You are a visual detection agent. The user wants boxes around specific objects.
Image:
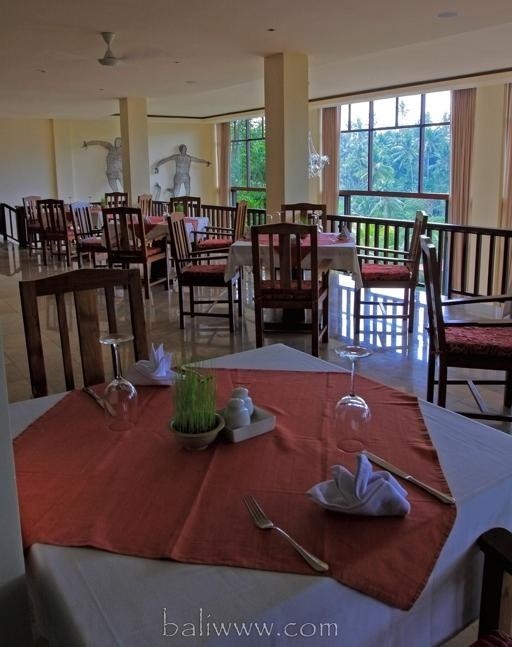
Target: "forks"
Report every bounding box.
[242,494,329,573]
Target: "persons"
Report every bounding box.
[153,144,214,198]
[80,136,124,193]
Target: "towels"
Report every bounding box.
[304,453,410,517]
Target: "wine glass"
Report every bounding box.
[333,345,371,452]
[311,215,321,238]
[314,211,323,233]
[98,333,138,432]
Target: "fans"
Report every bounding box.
[71,31,158,66]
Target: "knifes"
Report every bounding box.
[83,386,116,416]
[364,450,455,505]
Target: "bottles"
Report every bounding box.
[231,387,253,416]
[224,398,250,428]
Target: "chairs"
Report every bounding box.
[469,527,512,647]
